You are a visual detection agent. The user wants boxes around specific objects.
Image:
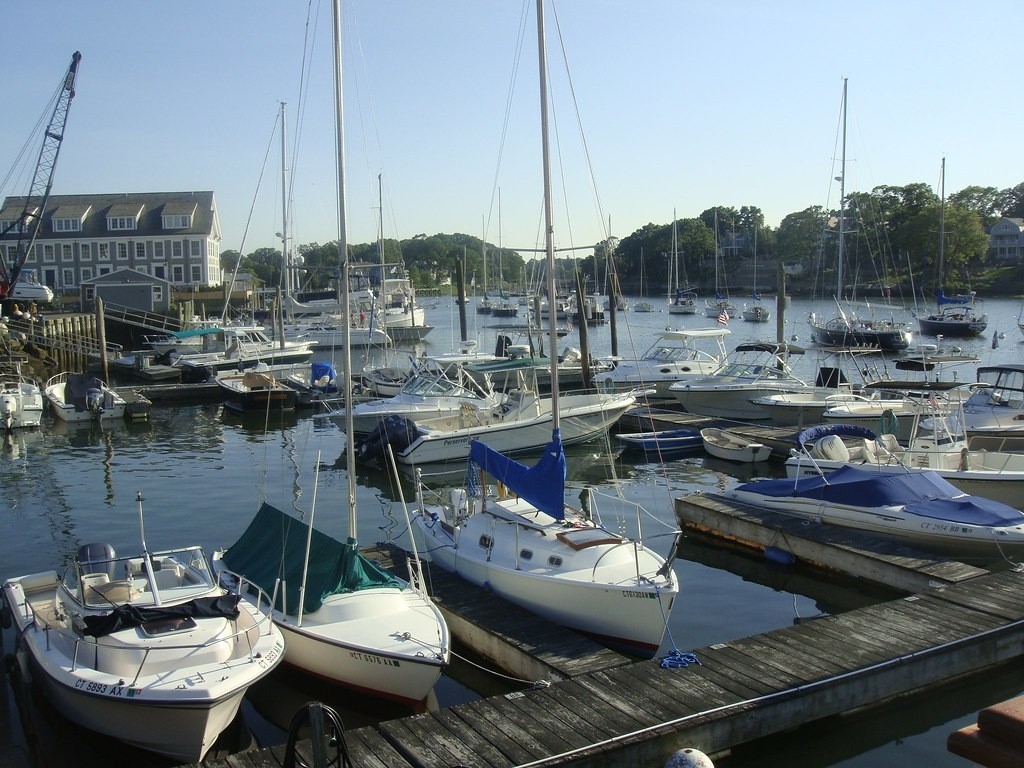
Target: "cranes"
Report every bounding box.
[0,50,83,319]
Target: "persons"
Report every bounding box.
[12,302,39,322]
[350,310,366,326]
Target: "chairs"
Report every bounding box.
[81,573,111,606]
[879,434,905,452]
[146,565,182,590]
[863,438,895,465]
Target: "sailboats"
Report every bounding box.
[139,1,1023,707]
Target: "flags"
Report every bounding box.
[718,310,729,326]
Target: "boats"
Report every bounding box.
[1,488,290,765]
[45,371,128,424]
[0,373,44,429]
